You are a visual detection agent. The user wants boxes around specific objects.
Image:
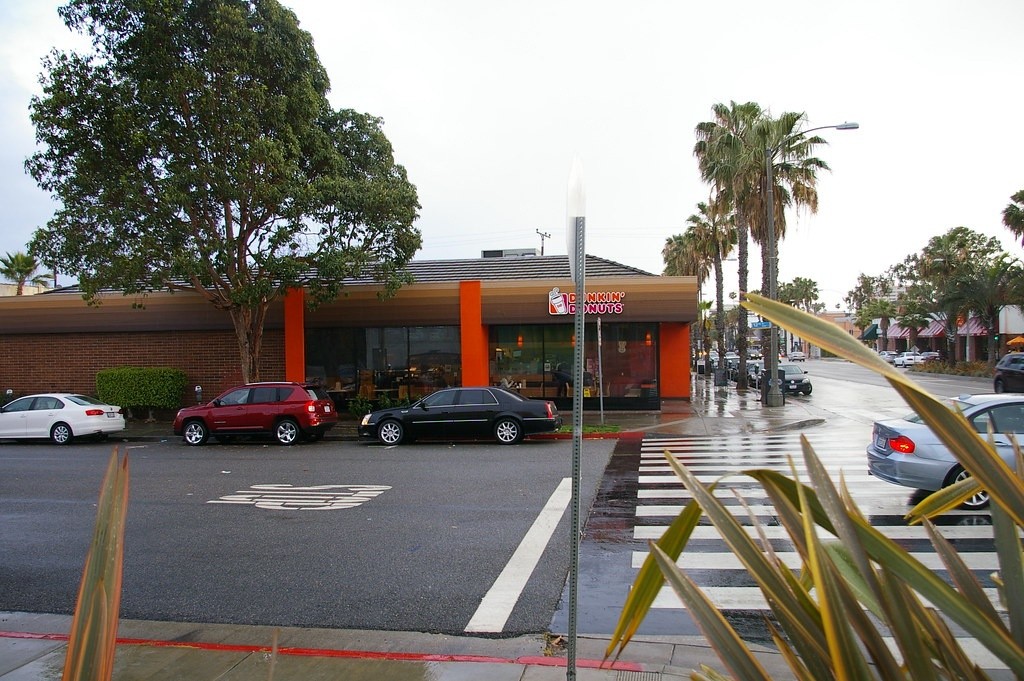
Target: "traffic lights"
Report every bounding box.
[994,335,1000,349]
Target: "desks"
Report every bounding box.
[511,386,526,395]
[372,388,398,392]
[326,389,355,411]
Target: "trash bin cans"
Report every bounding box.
[698,365,704,374]
[714,366,727,385]
[761,369,785,405]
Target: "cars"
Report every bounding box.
[893,351,924,368]
[867,392,1024,510]
[357,386,563,445]
[709,351,765,388]
[778,363,812,396]
[526,370,592,387]
[920,352,945,363]
[746,349,762,360]
[0,393,126,445]
[880,351,898,363]
[788,352,805,362]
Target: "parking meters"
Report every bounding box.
[6,389,13,404]
[194,385,203,405]
[754,363,759,396]
[728,360,732,387]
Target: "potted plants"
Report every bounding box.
[348,393,372,423]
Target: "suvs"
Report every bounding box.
[173,381,338,446]
[993,352,1024,393]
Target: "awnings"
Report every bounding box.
[887,323,923,339]
[957,317,998,337]
[918,320,946,337]
[857,324,878,339]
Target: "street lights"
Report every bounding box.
[934,258,970,361]
[766,123,860,409]
[700,258,738,352]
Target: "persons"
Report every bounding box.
[1008,347,1024,353]
[555,363,569,397]
[500,374,514,388]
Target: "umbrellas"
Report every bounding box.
[1006,336,1024,346]
